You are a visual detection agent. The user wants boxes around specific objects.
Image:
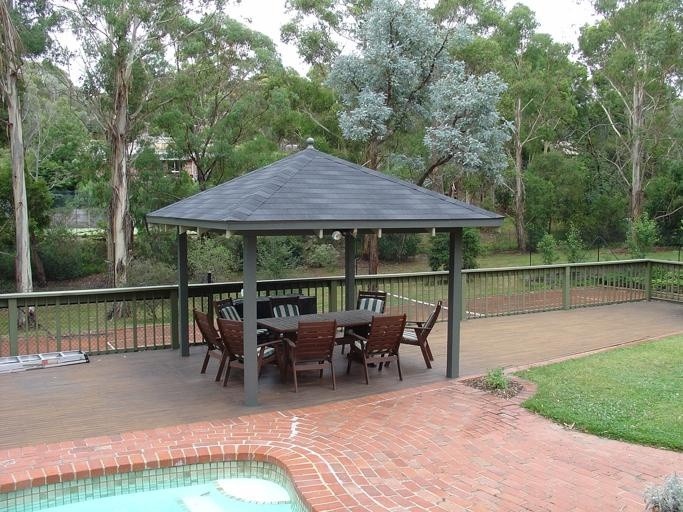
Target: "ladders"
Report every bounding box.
[0,350,89,373]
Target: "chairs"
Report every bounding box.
[191,290,441,394]
